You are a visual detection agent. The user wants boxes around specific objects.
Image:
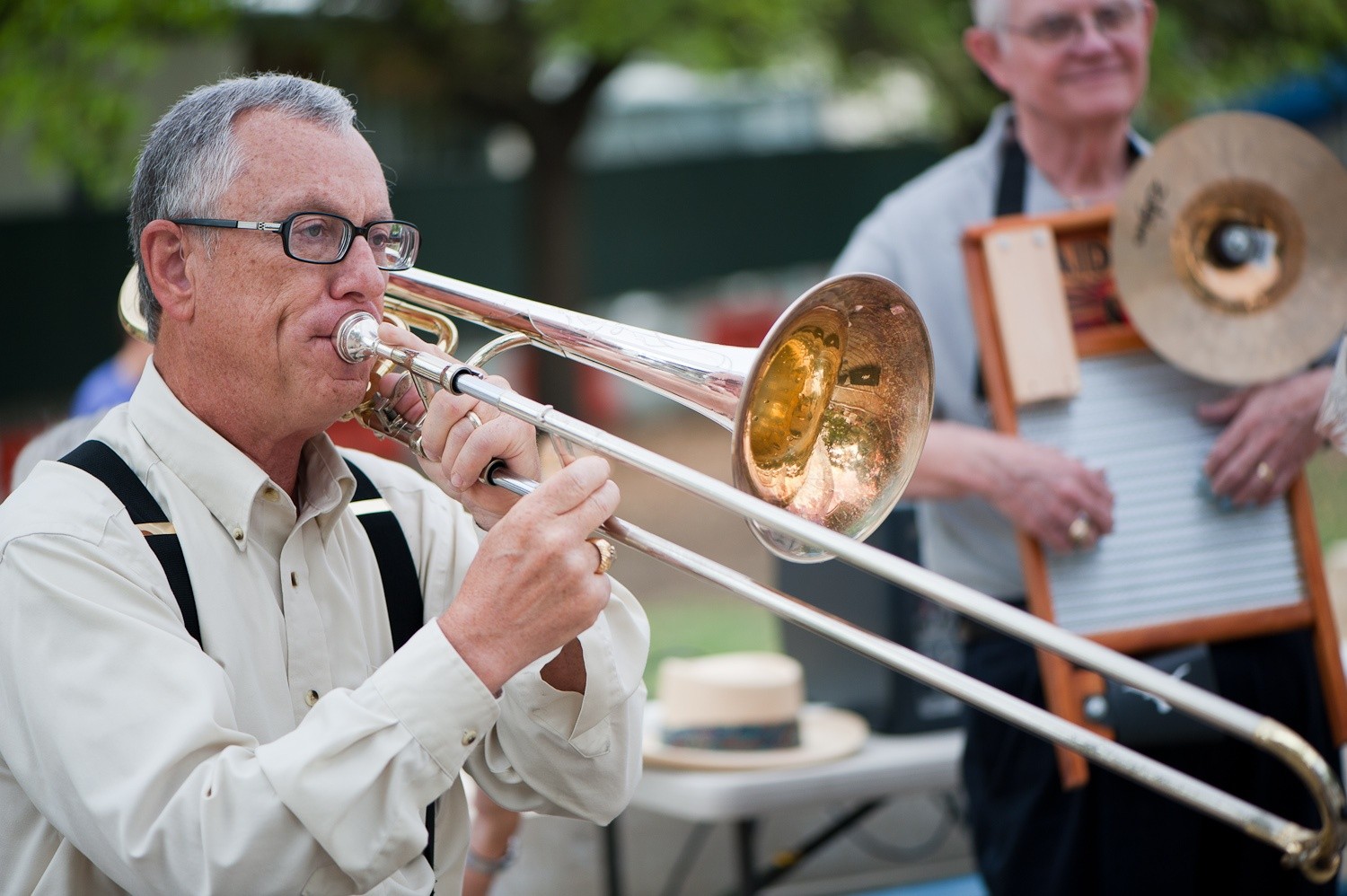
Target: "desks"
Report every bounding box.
[601,726,976,896]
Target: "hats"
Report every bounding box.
[637,648,873,775]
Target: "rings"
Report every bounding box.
[1255,461,1277,486]
[1065,509,1092,545]
[586,535,617,577]
[463,411,485,430]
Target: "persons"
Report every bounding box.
[2,75,652,894]
[69,329,155,421]
[810,3,1347,896]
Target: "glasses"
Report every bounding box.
[989,4,1145,44]
[159,210,420,273]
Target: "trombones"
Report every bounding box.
[117,245,1347,882]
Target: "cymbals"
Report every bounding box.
[1111,110,1347,385]
